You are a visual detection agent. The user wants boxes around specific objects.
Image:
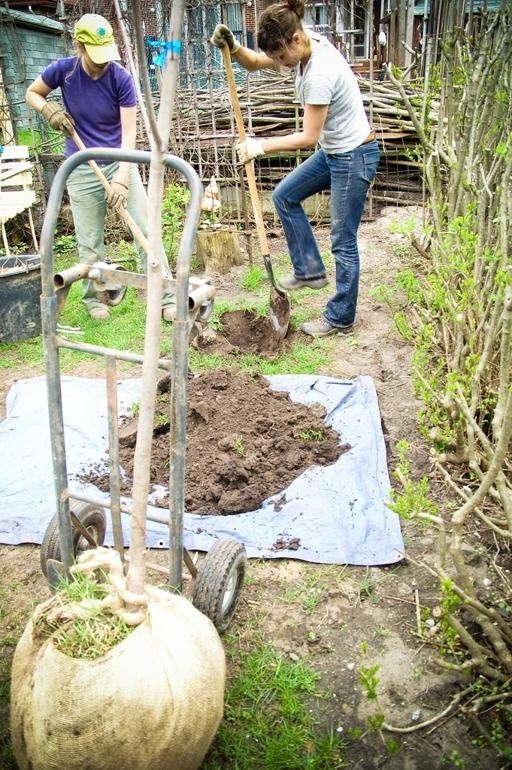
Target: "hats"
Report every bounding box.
[74,13,122,64]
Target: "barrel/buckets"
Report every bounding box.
[0,255,42,343]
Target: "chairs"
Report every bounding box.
[0,146,38,254]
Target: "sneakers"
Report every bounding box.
[163,307,177,322]
[89,306,109,320]
[300,317,353,337]
[279,275,328,290]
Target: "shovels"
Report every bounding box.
[220,40,290,340]
[64,117,217,350]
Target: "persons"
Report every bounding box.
[24,14,177,323]
[209,2,381,339]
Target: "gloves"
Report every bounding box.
[104,173,129,210]
[40,100,76,139]
[210,23,241,55]
[236,136,266,165]
